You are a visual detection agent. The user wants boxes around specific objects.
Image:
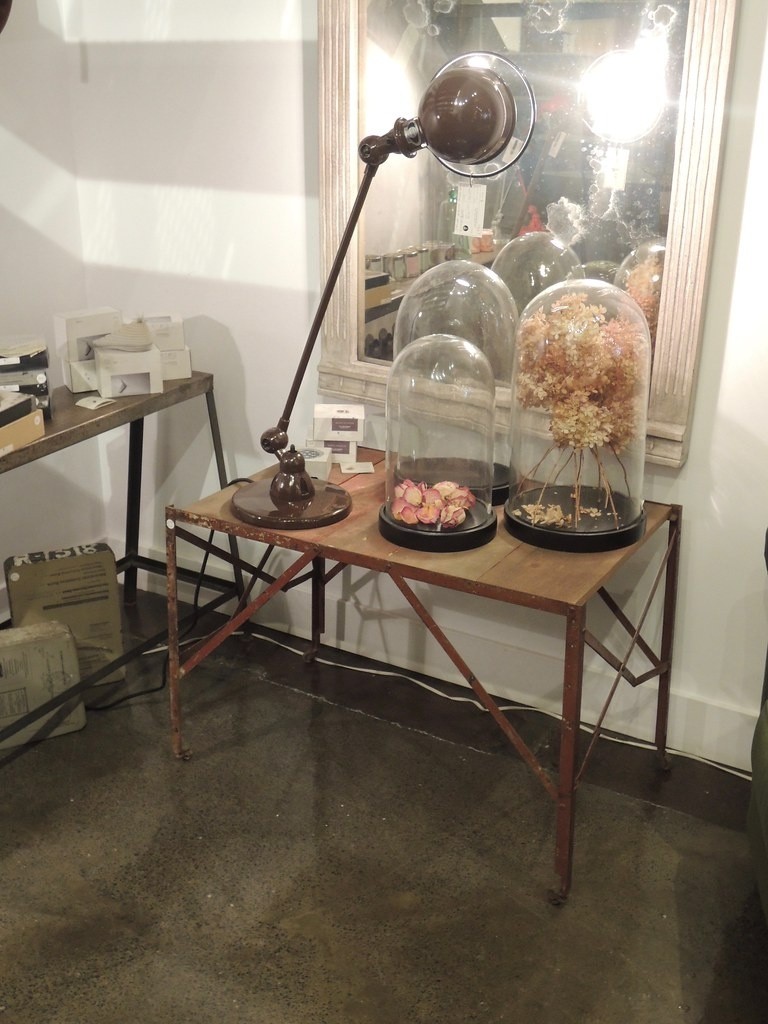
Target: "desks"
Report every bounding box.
[165,446,683,899]
[0,371,251,744]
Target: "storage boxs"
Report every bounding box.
[306,438,357,463]
[94,348,164,399]
[0,620,88,750]
[61,358,98,393]
[311,404,365,443]
[160,346,194,381]
[0,409,46,459]
[53,306,124,361]
[145,314,186,351]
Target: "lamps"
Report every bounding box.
[229,53,535,532]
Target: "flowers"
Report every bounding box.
[391,479,479,528]
[518,295,647,531]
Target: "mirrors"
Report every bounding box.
[311,0,742,468]
